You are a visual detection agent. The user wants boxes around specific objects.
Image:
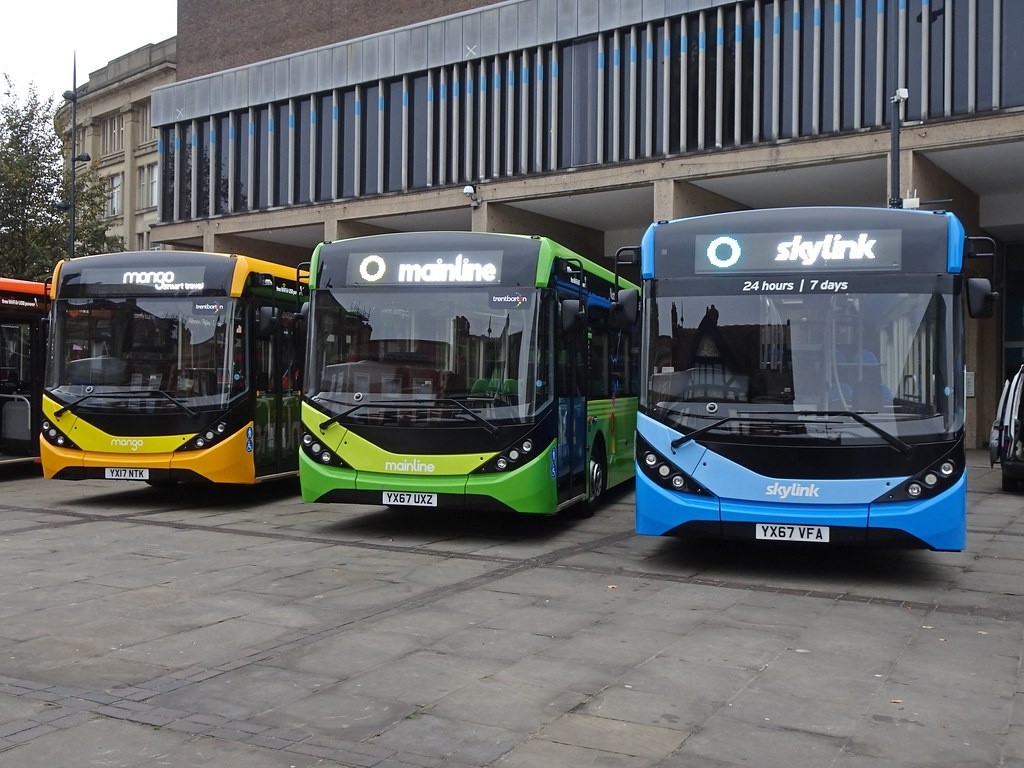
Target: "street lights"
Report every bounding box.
[58,89,90,258]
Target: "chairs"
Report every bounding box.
[819,343,894,409]
[471,378,622,400]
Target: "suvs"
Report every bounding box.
[990,362,1024,490]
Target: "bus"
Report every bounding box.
[289,228,642,521]
[0,274,56,467]
[609,206,995,558]
[38,246,320,499]
